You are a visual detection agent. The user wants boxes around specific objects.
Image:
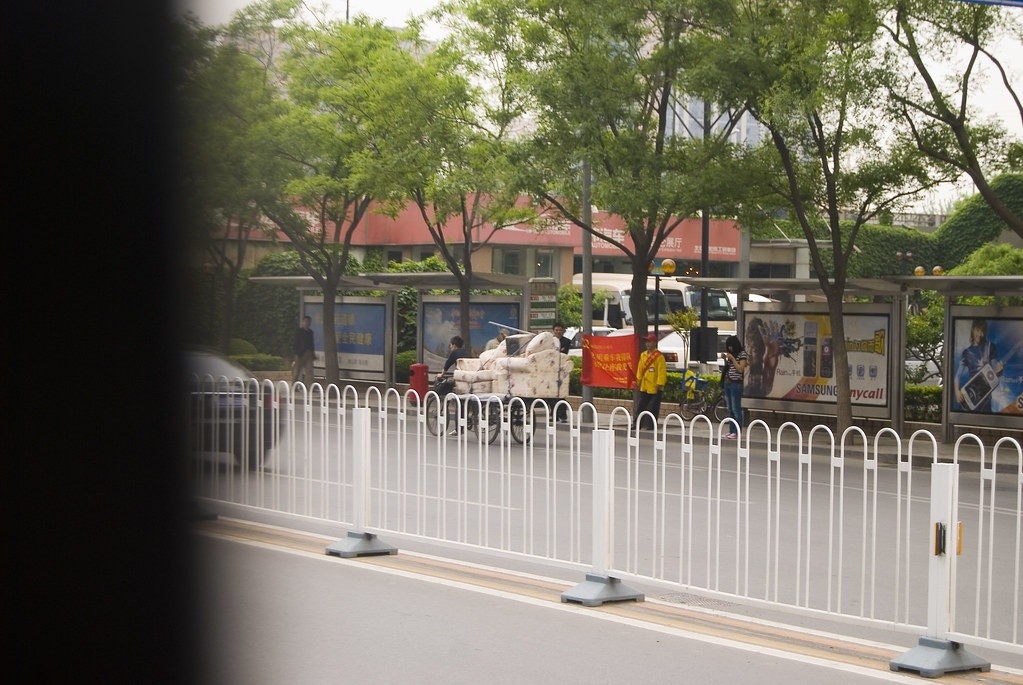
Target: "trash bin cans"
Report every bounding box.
[409,363,429,407]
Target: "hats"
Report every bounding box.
[642,333,657,341]
[500,328,510,337]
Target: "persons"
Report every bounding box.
[437,336,469,435]
[485,329,510,351]
[291,316,318,393]
[627,335,666,430]
[549,323,571,423]
[745,317,790,398]
[954,319,1004,414]
[721,336,747,439]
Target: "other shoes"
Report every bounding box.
[646,426,654,430]
[727,433,737,439]
[549,417,559,422]
[722,432,731,438]
[631,426,641,430]
[561,419,567,423]
[448,430,464,435]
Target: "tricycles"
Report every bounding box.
[427,376,537,444]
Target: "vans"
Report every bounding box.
[570,282,627,334]
[718,288,771,312]
[571,272,738,372]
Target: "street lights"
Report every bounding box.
[648,258,676,352]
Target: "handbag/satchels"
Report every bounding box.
[721,366,728,389]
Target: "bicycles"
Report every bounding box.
[679,378,738,426]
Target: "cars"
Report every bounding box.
[197,351,285,467]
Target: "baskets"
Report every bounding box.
[433,376,453,395]
